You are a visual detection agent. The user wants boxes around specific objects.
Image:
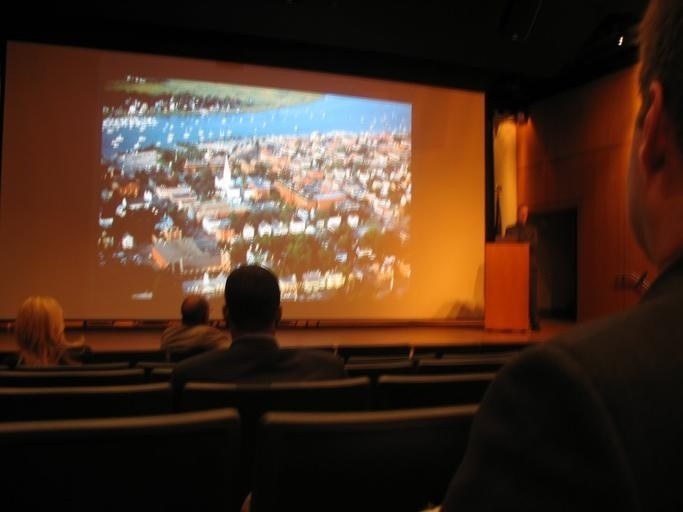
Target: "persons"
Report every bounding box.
[169,264,346,397]
[504,205,540,330]
[159,294,230,363]
[1,295,84,371]
[435,1,682,512]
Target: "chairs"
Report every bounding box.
[0,341,543,512]
